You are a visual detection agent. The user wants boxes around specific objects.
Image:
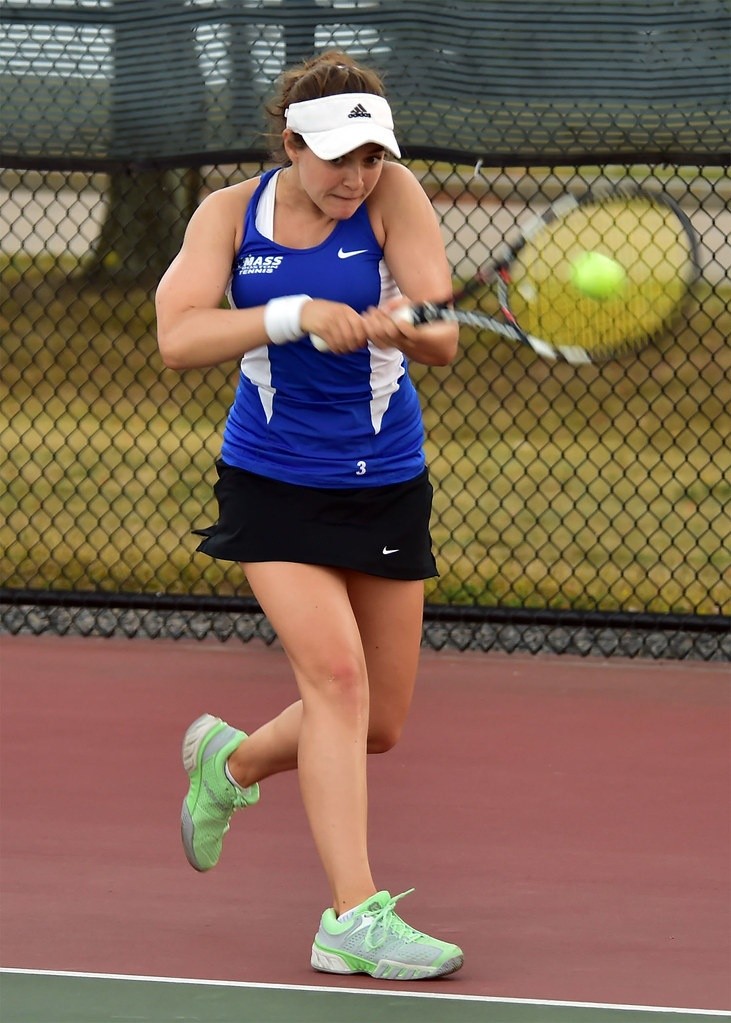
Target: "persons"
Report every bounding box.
[156,52,464,981]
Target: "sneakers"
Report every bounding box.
[181,712,261,872]
[311,888,464,981]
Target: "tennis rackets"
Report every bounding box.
[309,184,702,367]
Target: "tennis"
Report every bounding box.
[570,252,626,301]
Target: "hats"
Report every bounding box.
[284,93,401,160]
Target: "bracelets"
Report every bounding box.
[264,294,314,344]
